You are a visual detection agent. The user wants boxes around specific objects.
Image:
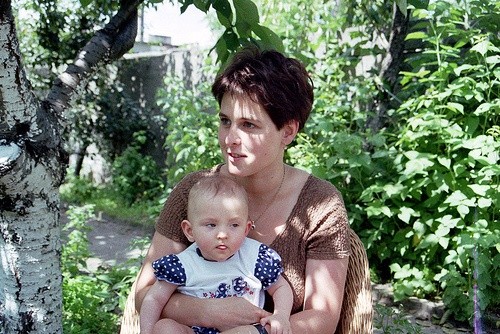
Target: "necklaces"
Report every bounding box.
[248,164,285,231]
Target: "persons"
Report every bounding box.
[133,43,352,334]
[139,174,293,334]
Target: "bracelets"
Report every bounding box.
[251,322,268,334]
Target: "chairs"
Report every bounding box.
[120,228,374,334]
